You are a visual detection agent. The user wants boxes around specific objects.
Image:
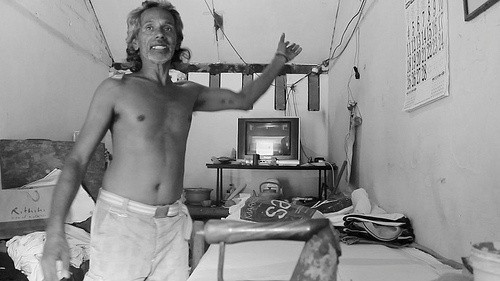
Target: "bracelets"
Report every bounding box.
[276,52,289,63]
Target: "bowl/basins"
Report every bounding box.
[185,187,213,205]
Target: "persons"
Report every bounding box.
[40,1,304,281]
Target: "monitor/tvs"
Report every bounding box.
[238,117,301,165]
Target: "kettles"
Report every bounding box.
[252,181,284,202]
